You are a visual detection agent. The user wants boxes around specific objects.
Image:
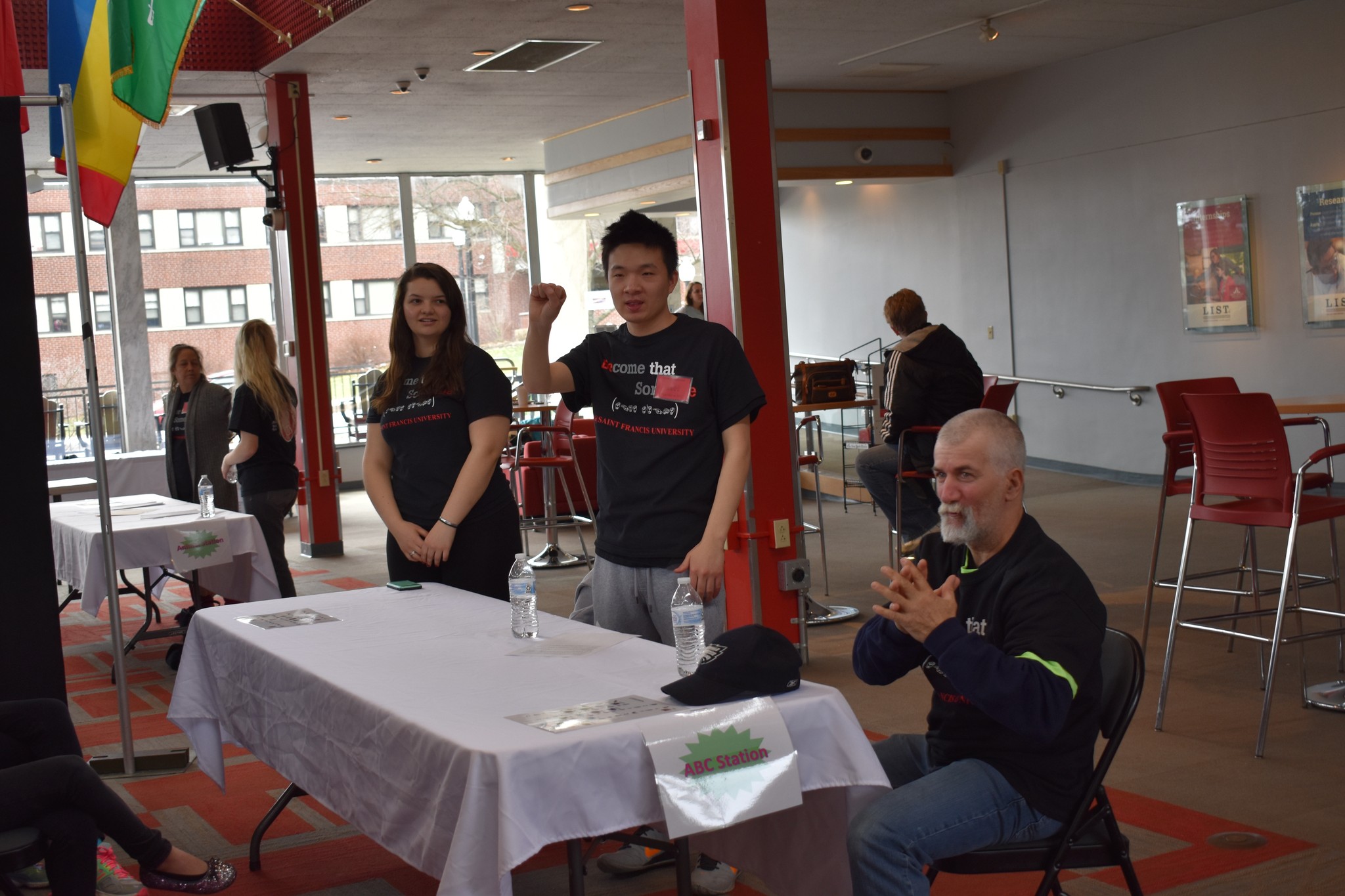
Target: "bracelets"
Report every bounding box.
[439,516,457,528]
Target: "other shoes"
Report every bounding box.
[139,857,237,894]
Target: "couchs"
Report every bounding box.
[503,419,597,517]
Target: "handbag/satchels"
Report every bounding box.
[788,358,859,405]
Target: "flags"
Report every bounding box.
[0,0,202,230]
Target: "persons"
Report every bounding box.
[162,343,238,625]
[520,208,766,895]
[362,263,524,603]
[1195,248,1246,303]
[673,282,705,320]
[849,408,1109,896]
[0,699,238,896]
[1306,237,1345,296]
[221,320,300,600]
[854,288,984,553]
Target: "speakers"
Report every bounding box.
[194,103,254,172]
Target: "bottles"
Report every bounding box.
[226,464,238,484]
[198,475,216,518]
[671,577,706,677]
[508,553,540,639]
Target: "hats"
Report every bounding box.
[658,623,802,706]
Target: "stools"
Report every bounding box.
[797,375,1021,595]
[1142,377,1345,691]
[1154,392,1345,759]
[501,398,598,572]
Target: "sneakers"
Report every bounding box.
[91,842,142,896]
[689,851,740,894]
[595,824,678,875]
[5,859,50,889]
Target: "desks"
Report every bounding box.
[510,403,595,570]
[793,393,878,625]
[1272,397,1345,710]
[47,476,97,503]
[49,493,283,685]
[164,580,893,896]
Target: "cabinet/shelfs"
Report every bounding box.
[839,337,903,516]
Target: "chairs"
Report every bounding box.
[42,396,70,459]
[341,369,382,443]
[73,389,122,458]
[926,626,1146,896]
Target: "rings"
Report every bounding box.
[410,551,416,555]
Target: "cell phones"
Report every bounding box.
[386,580,422,591]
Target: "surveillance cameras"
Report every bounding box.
[414,68,429,80]
[855,147,874,163]
[263,208,284,231]
[395,81,411,92]
